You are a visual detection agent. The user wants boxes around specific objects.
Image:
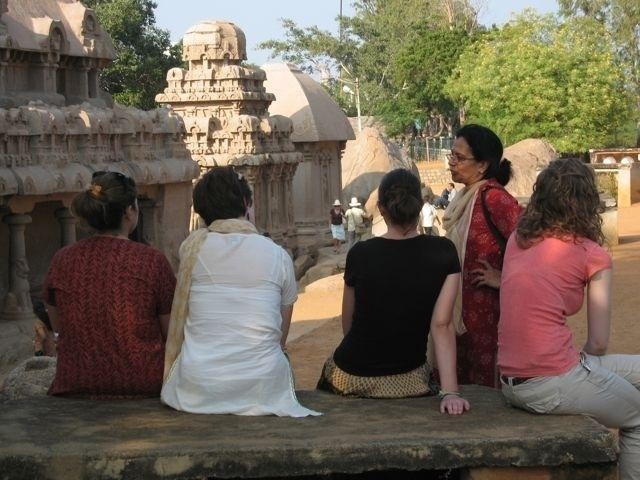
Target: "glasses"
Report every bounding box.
[92,171,126,192]
[446,154,475,161]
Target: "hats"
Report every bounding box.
[349,198,361,206]
[332,200,342,206]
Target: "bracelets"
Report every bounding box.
[438,389,462,397]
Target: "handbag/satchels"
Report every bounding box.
[355,223,366,234]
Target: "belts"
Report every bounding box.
[501,376,533,386]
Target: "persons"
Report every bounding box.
[419,195,441,235]
[340,197,372,249]
[315,167,471,418]
[329,199,346,254]
[158,163,299,414]
[496,156,640,480]
[435,182,457,209]
[42,172,178,401]
[441,121,527,390]
